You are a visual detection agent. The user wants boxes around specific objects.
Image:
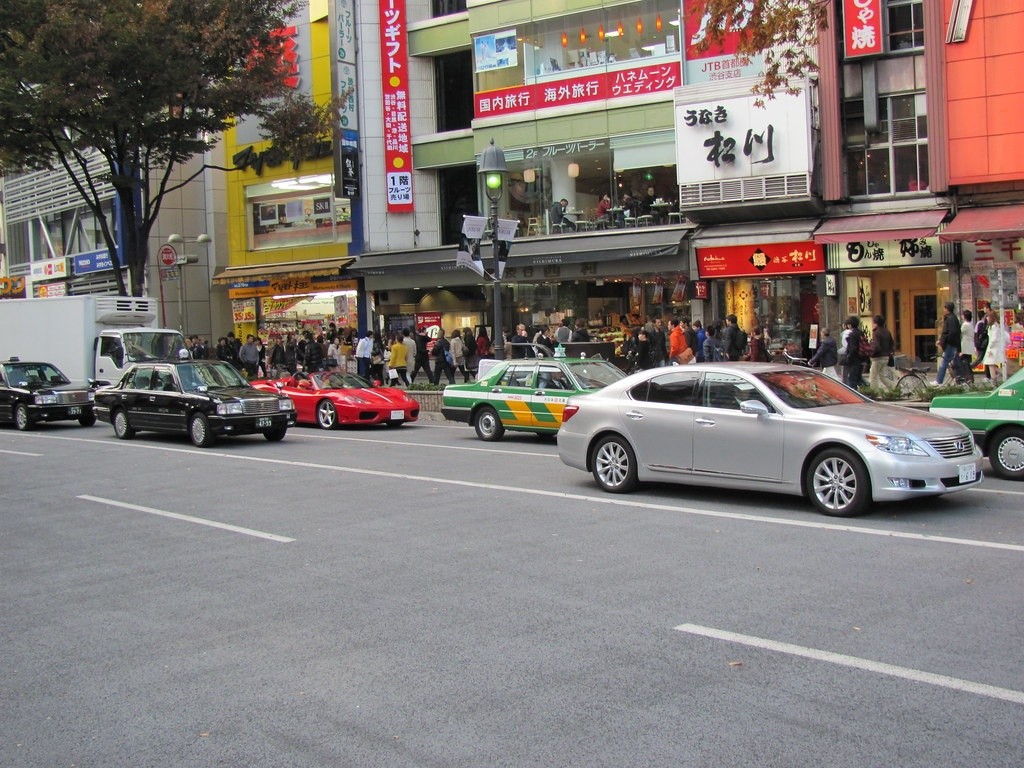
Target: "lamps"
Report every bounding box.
[524,169,535,183]
[567,158,579,177]
[559,0,663,47]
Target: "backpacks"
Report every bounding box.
[708,341,726,361]
[757,342,773,362]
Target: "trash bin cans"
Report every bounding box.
[560,340,616,364]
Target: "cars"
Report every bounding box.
[928,366,1024,481]
[440,356,643,442]
[556,360,983,519]
[94,359,298,448]
[0,357,97,431]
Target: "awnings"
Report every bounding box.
[814,210,949,244]
[211,258,353,285]
[346,229,688,277]
[935,204,1024,245]
[691,219,820,247]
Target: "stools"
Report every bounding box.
[527,217,540,236]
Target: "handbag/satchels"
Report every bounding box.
[388,367,398,379]
[839,353,850,365]
[444,350,452,362]
[466,355,482,369]
[887,353,894,367]
[678,348,694,364]
[257,366,264,378]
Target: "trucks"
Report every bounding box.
[0,294,194,391]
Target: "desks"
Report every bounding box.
[607,207,624,228]
[650,202,672,225]
[562,212,586,231]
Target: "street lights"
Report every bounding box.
[478,136,512,355]
[167,234,213,336]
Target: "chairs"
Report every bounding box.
[159,375,172,390]
[538,372,552,390]
[545,208,684,235]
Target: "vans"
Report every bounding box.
[489,342,554,359]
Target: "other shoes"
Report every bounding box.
[929,380,943,386]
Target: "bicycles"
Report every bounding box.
[894,350,979,403]
[781,347,818,368]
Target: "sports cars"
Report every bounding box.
[248,369,421,430]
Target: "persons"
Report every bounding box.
[595,192,611,229]
[362,327,490,387]
[807,314,907,392]
[544,372,568,390]
[617,190,634,225]
[185,335,210,360]
[930,302,1009,387]
[551,199,580,232]
[623,314,767,375]
[640,186,665,224]
[216,323,359,381]
[298,379,309,389]
[493,318,589,360]
